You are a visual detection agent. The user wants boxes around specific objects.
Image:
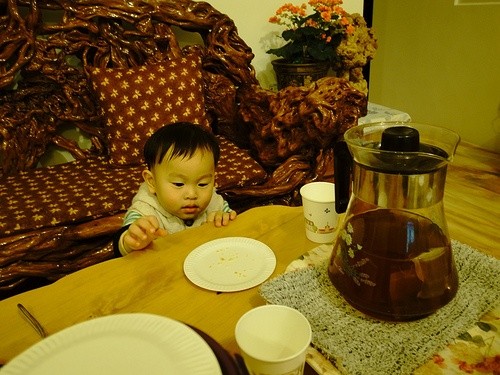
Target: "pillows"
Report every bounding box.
[85,55,212,169]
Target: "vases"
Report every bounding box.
[271,59,330,92]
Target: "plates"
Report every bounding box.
[0,312,223,375]
[183,236,276,292]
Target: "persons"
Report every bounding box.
[113,122,237,260]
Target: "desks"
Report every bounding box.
[0,205,500,375]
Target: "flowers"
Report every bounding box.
[266,1,355,65]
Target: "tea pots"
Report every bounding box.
[326,121,461,321]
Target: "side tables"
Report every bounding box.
[322,101,411,177]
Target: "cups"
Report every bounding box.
[234,305,312,375]
[299,182,341,244]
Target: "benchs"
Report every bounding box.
[0,0,368,292]
